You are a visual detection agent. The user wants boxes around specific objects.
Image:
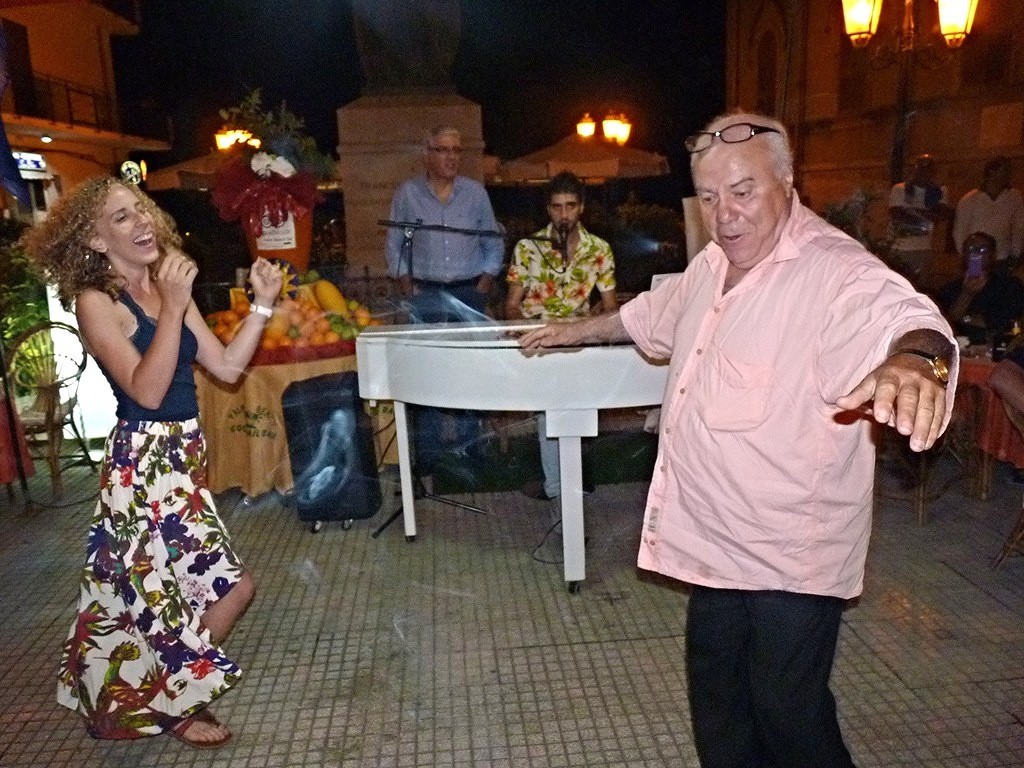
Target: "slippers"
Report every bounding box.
[165,710,233,749]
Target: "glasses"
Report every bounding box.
[967,246,987,254]
[684,123,780,160]
[430,146,461,154]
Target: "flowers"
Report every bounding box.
[211,95,334,237]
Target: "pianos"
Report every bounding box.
[354,324,669,579]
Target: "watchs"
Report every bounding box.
[963,315,973,323]
[892,349,950,386]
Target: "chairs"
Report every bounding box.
[0,321,97,503]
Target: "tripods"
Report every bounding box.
[372,219,561,538]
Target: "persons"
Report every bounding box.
[19,175,283,748]
[886,154,951,276]
[505,168,618,536]
[942,234,1024,488]
[514,112,959,768]
[384,122,504,499]
[951,155,1022,266]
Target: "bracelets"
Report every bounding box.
[248,305,275,319]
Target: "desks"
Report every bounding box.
[913,345,1024,523]
[194,323,390,500]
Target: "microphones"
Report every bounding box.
[559,223,568,271]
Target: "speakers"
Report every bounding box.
[281,370,384,523]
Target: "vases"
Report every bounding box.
[243,195,315,273]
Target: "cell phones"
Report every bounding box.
[969,253,984,279]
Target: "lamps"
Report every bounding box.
[936,0,979,44]
[841,0,883,46]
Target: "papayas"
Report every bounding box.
[294,280,347,316]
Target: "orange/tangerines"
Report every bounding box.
[204,300,382,349]
